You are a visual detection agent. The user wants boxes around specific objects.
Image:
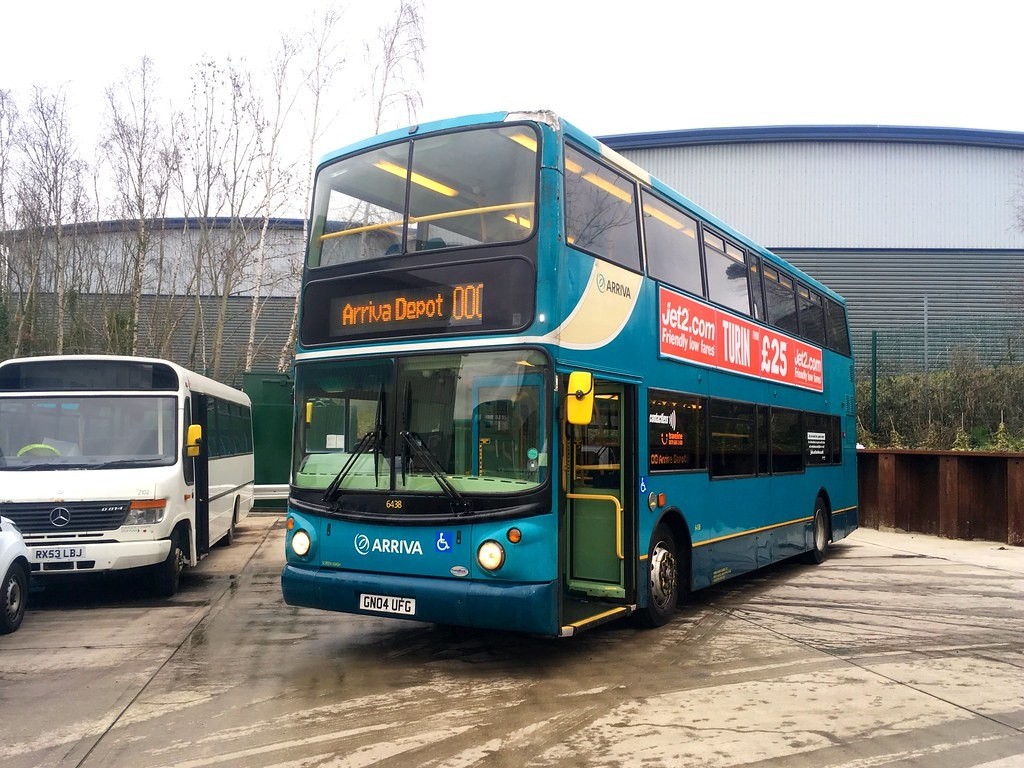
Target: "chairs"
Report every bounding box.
[214,437,226,455]
[235,430,248,453]
[208,436,219,456]
[223,436,238,454]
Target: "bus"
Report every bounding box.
[280,109,860,639]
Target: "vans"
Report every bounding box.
[0,355,255,597]
